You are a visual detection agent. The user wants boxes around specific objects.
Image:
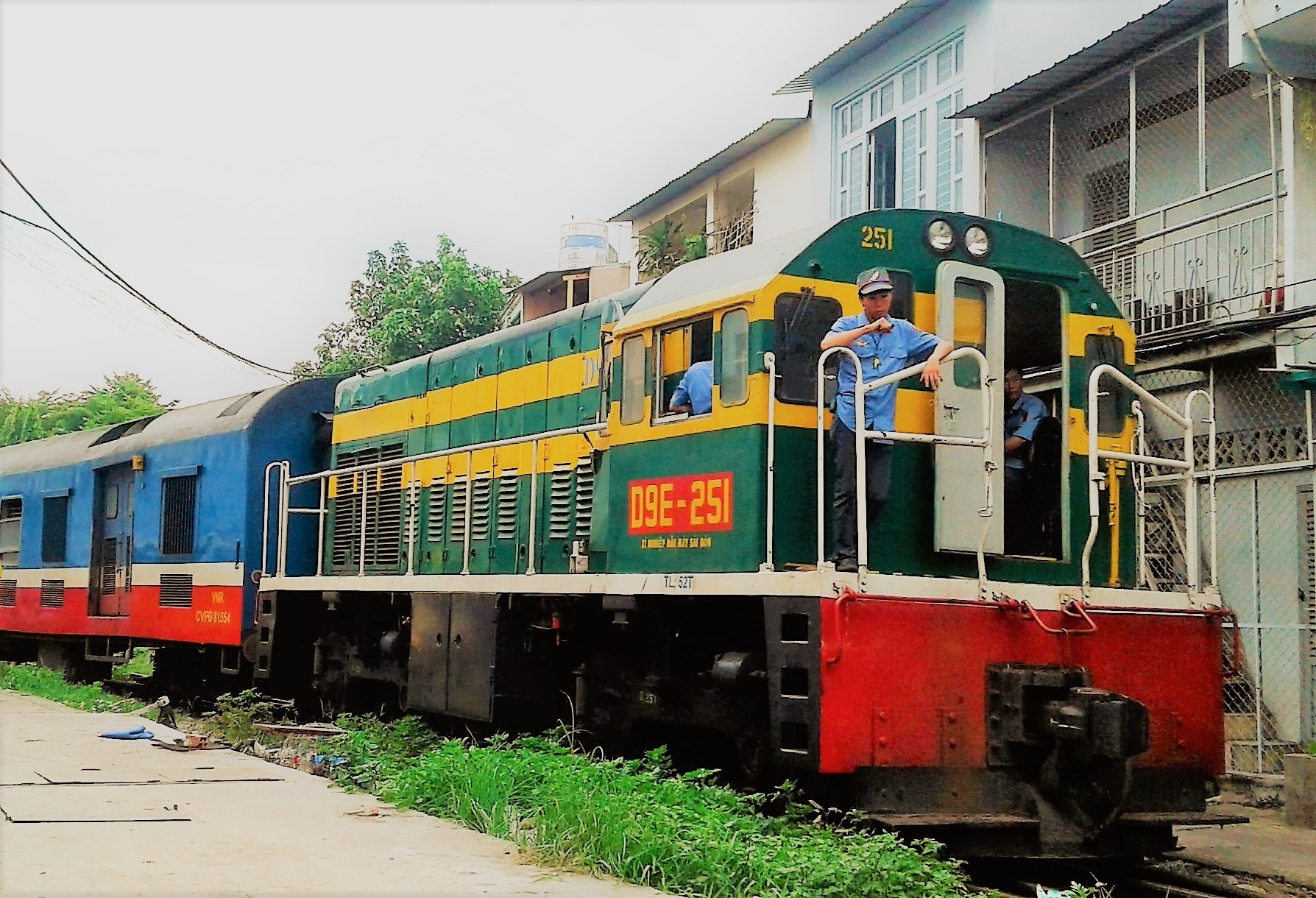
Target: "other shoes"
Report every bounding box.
[837,558,859,572]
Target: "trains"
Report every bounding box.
[0,208,1251,881]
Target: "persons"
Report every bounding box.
[669,359,713,415]
[820,266,954,572]
[1004,367,1048,502]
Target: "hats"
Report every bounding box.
[858,265,893,294]
